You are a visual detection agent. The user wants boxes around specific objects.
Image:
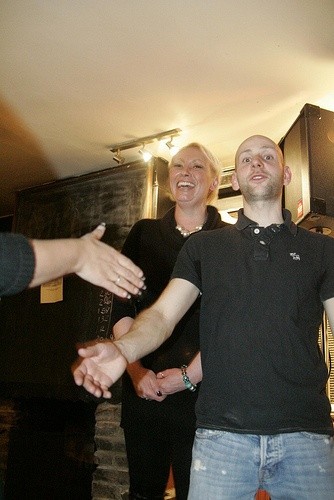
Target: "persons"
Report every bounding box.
[73,136,334,500]
[0,223,145,299]
[111,141,236,500]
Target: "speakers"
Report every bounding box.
[278,103,334,239]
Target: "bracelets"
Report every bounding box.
[182,365,197,392]
[111,340,129,365]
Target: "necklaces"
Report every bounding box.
[176,224,203,238]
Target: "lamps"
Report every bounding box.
[110,129,182,164]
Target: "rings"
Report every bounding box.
[155,389,162,396]
[160,372,165,379]
